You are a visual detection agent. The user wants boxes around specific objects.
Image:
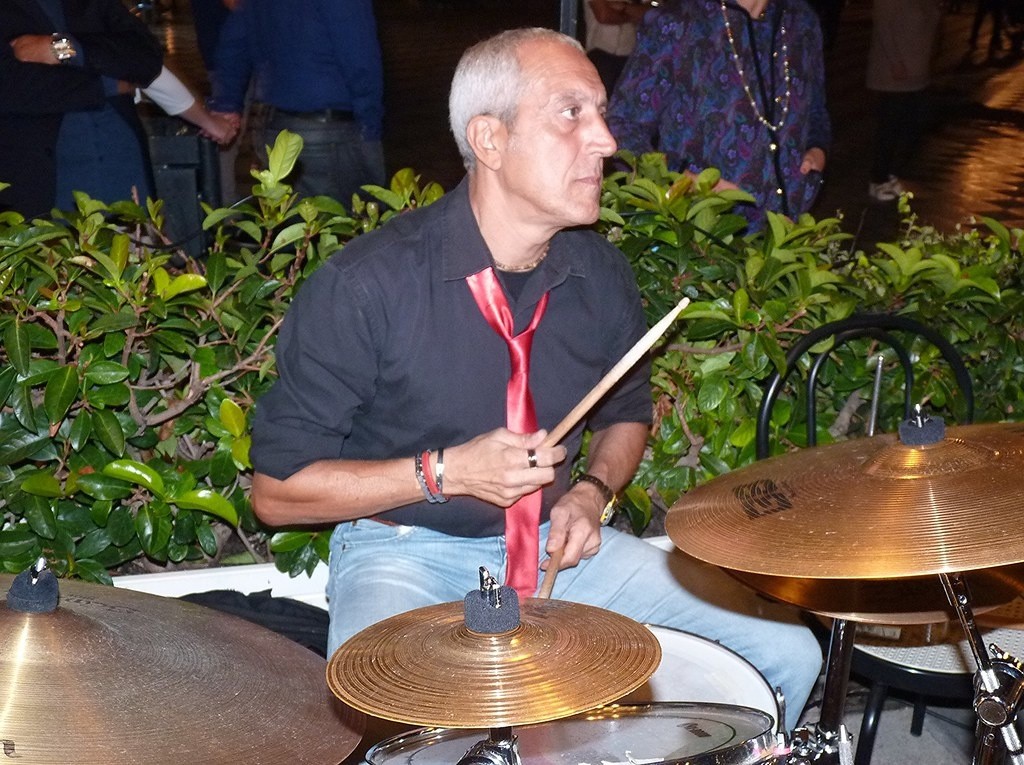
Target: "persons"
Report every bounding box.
[0,0,387,270]
[248,27,824,764]
[578,0,945,239]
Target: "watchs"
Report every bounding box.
[49,33,72,66]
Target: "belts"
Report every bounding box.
[276,108,355,123]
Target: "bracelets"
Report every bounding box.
[415,448,449,504]
[573,474,618,528]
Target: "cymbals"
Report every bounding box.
[2,568,371,765]
[324,596,662,730]
[661,420,1024,582]
[718,566,1024,625]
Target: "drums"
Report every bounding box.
[608,620,782,739]
[365,700,778,765]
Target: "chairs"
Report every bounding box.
[755,311,1024,765]
[148,135,241,267]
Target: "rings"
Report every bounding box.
[528,449,536,468]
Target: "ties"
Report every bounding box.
[467,268,542,600]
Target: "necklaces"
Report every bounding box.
[494,239,550,271]
[721,1,791,131]
